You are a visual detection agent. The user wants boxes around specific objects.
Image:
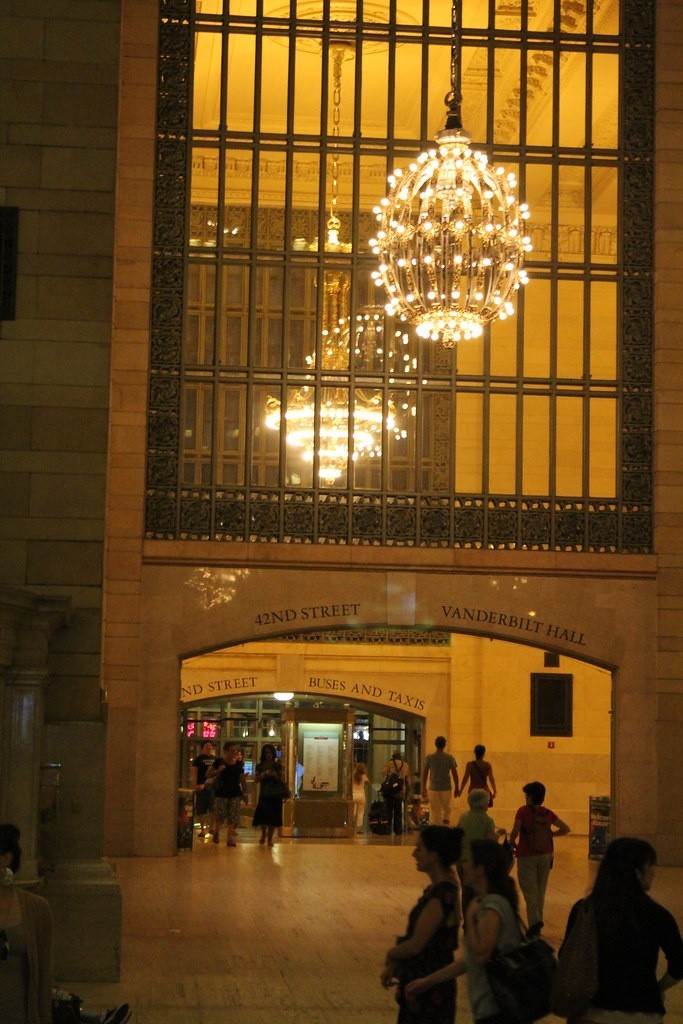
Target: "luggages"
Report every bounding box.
[368,789,391,834]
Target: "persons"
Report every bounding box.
[459,744,497,800]
[456,789,507,935]
[205,741,248,847]
[422,736,459,827]
[352,762,370,834]
[559,837,683,1024]
[407,837,524,1024]
[510,781,570,934]
[383,750,412,835]
[381,825,465,1024]
[0,823,56,1024]
[192,739,218,837]
[252,744,283,847]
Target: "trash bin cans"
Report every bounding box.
[40,763,61,873]
[587,796,611,859]
[179,788,196,850]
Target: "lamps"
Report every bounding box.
[262,0,418,487]
[368,0,535,349]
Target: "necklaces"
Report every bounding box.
[4,891,14,922]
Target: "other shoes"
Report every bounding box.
[268,839,273,846]
[213,835,219,844]
[227,841,236,847]
[103,1003,132,1024]
[526,920,543,939]
[209,829,214,835]
[260,837,266,844]
[198,832,205,837]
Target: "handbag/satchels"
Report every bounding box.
[269,776,290,799]
[203,758,223,792]
[501,831,514,875]
[383,773,406,800]
[489,792,494,807]
[473,895,561,1024]
[549,898,600,1020]
